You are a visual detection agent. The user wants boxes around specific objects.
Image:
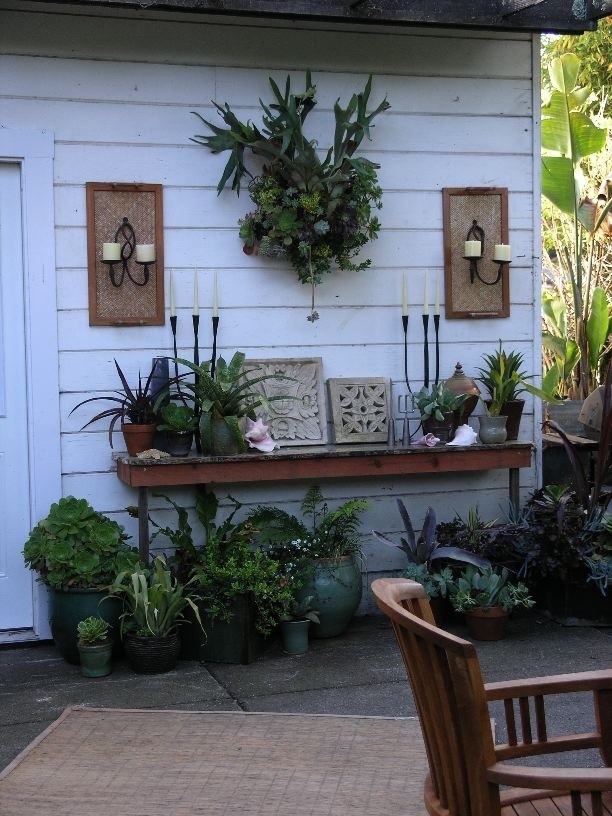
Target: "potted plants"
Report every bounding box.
[67,351,304,456]
[19,483,370,678]
[371,338,612,641]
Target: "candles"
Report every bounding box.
[402,271,408,316]
[434,269,440,315]
[465,240,481,257]
[424,269,429,315]
[213,272,218,318]
[102,243,121,260]
[136,242,156,263]
[170,270,176,317]
[193,268,199,315]
[494,242,511,261]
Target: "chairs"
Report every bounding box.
[371,577,612,816]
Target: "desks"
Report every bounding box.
[110,439,533,565]
